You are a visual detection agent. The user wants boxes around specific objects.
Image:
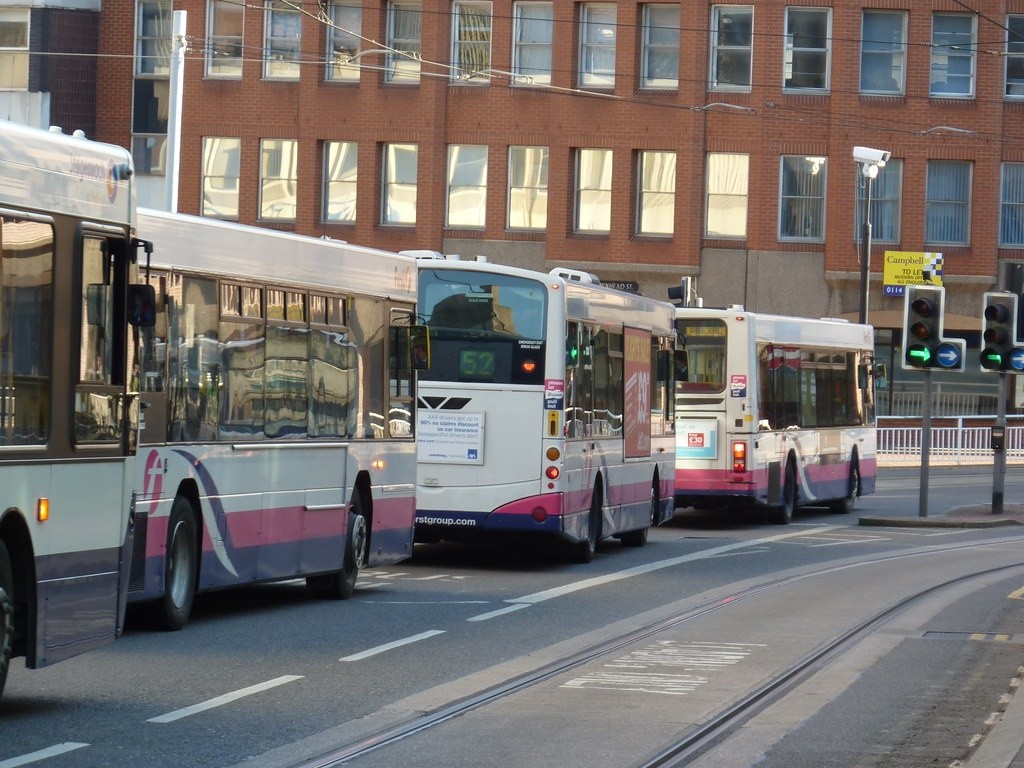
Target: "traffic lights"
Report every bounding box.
[583,326,592,370]
[902,284,967,374]
[565,320,581,370]
[980,293,1024,375]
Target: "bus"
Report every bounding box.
[382,250,677,562]
[1,209,431,636]
[0,119,155,690]
[672,304,888,526]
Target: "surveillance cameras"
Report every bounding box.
[852,146,891,167]
